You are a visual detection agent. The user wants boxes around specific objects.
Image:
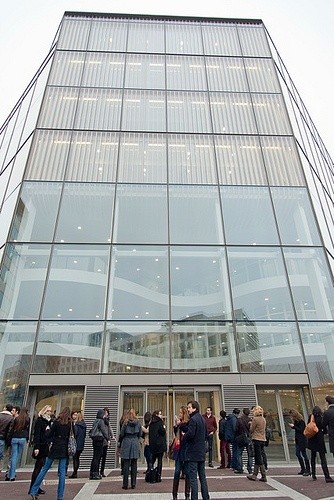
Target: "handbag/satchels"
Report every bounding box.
[145,467,161,483]
[88,420,104,441]
[173,438,180,450]
[168,439,175,459]
[236,435,248,448]
[67,421,77,456]
[304,414,318,438]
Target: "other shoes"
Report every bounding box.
[185,493,190,500]
[37,487,45,494]
[209,464,214,467]
[234,470,243,474]
[325,478,334,483]
[172,492,177,500]
[100,474,106,477]
[90,475,101,480]
[248,470,253,474]
[312,475,317,481]
[217,466,225,469]
[5,477,16,481]
[68,473,77,478]
[122,486,128,489]
[131,485,136,489]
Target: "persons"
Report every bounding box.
[0,404,30,480]
[202,407,217,467]
[307,396,334,482]
[117,408,166,489]
[173,401,210,500]
[266,407,294,441]
[289,408,312,475]
[90,408,113,480]
[217,406,268,482]
[28,405,86,500]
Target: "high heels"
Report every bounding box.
[297,470,312,476]
[32,494,39,500]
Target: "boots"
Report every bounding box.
[259,465,267,482]
[247,465,259,481]
